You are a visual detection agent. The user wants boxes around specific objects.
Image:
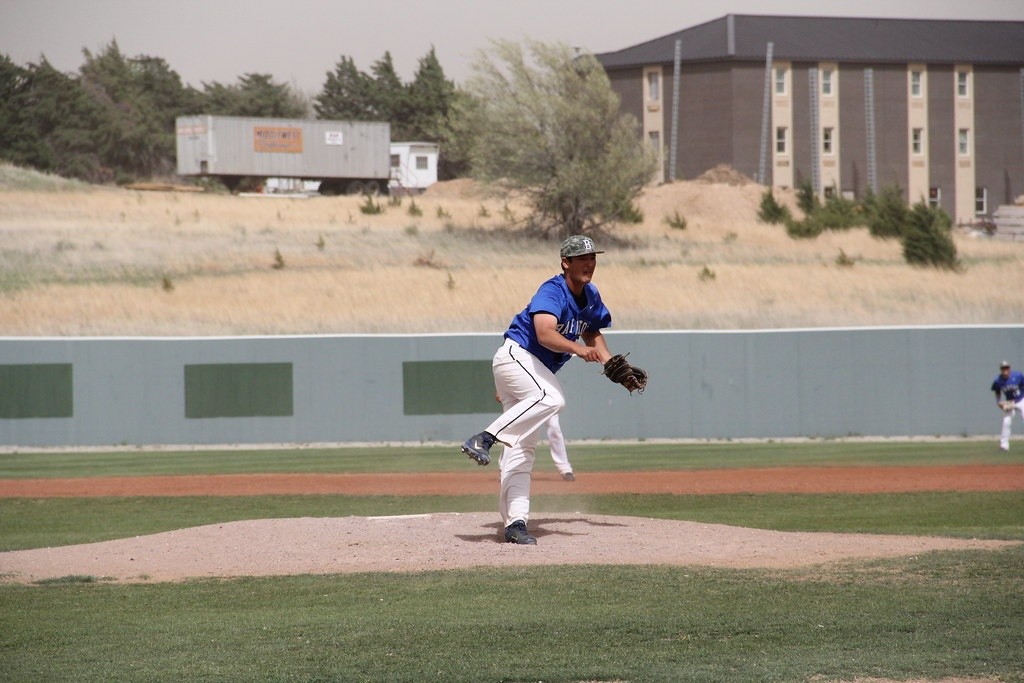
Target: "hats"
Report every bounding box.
[1000,361,1011,368]
[560,235,604,259]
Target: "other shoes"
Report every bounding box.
[564,473,577,482]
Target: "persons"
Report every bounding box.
[461,235,648,546]
[991,361,1024,451]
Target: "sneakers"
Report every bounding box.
[505,520,537,545]
[460,434,497,465]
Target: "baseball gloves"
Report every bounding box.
[604,354,648,392]
[999,400,1015,411]
